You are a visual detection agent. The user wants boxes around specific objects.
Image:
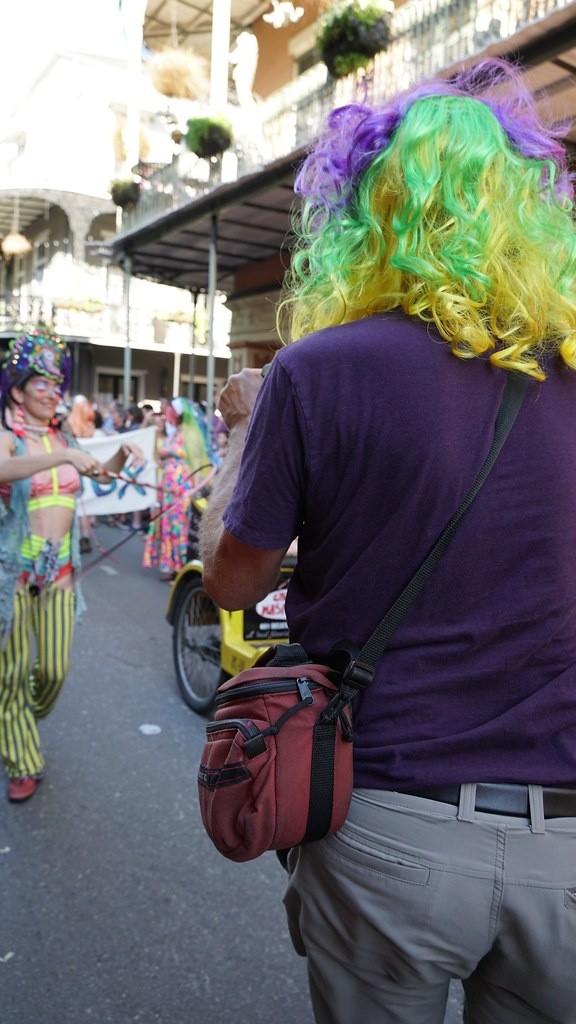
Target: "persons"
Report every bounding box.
[143,398,211,582]
[200,58,576,1024]
[0,371,148,801]
[59,393,173,553]
[196,400,230,457]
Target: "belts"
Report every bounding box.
[387,782,576,817]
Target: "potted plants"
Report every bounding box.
[187,116,233,157]
[314,0,394,77]
[109,179,141,211]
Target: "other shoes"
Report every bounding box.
[131,526,144,534]
[120,525,133,532]
[7,773,44,802]
[80,538,91,552]
[161,572,177,581]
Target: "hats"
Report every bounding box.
[0,326,72,407]
[171,397,185,415]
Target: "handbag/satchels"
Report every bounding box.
[198,643,374,862]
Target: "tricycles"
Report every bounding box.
[164,486,298,713]
